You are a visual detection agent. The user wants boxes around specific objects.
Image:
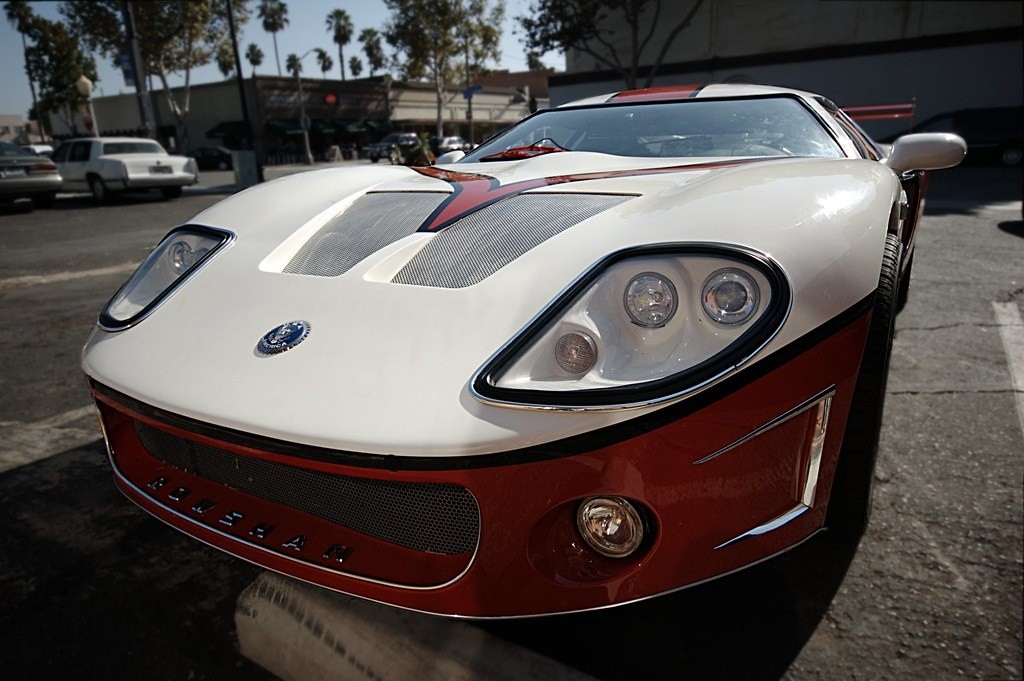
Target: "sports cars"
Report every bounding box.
[80,77,968,620]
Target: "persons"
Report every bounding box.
[346,142,357,160]
[406,143,422,166]
[372,144,396,162]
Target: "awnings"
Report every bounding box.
[206,121,246,138]
[390,107,530,123]
[159,126,176,136]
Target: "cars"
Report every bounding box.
[0,140,63,211]
[48,136,199,203]
[188,145,233,171]
[429,134,476,154]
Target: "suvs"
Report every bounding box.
[366,131,420,164]
